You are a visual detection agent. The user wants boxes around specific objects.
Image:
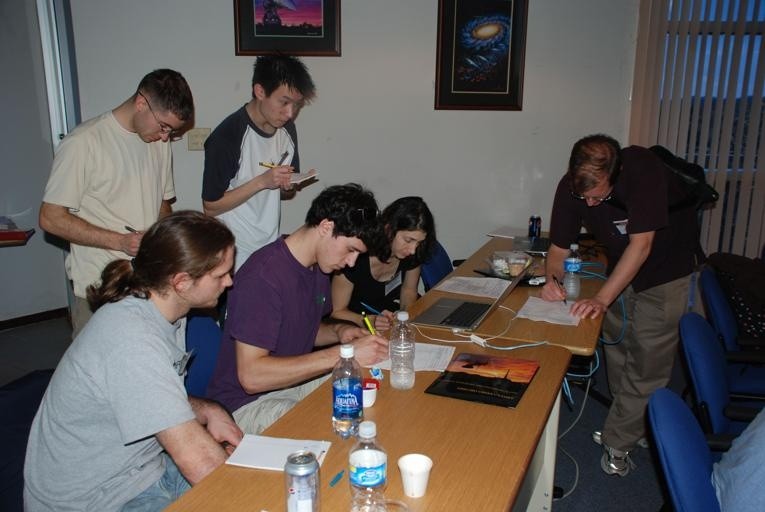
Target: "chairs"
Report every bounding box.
[420,237,466,292]
[184,315,223,397]
[647,387,765,511]
[698,263,765,354]
[678,312,764,439]
[0,368,57,512]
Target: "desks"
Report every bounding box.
[160,321,571,511]
[391,228,609,356]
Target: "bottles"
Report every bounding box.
[387,311,416,389]
[345,419,389,512]
[564,244,582,300]
[330,342,365,438]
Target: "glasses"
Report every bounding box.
[570,191,613,203]
[138,91,182,142]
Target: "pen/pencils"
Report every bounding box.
[125,225,139,235]
[360,301,384,316]
[362,311,376,335]
[258,162,278,168]
[327,469,345,490]
[551,274,567,305]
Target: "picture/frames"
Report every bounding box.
[434,0,530,111]
[232,0,342,57]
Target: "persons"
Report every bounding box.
[541,132,697,479]
[206,181,392,436]
[22,211,243,512]
[202,51,319,275]
[39,69,194,341]
[330,196,435,331]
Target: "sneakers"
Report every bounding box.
[593,430,656,478]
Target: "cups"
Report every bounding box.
[397,451,434,499]
[363,388,376,408]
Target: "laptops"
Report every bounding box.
[510,235,548,258]
[410,263,533,331]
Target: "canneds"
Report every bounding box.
[528,215,541,244]
[283,450,321,512]
